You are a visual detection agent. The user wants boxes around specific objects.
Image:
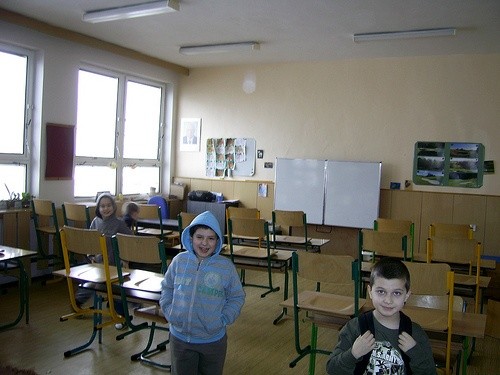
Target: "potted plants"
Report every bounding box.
[21,193,32,208]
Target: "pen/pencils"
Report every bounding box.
[197,262,200,271]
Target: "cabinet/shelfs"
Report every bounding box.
[188,200,239,233]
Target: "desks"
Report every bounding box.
[173,243,297,324]
[360,262,491,366]
[138,219,179,248]
[363,250,496,315]
[280,290,486,375]
[225,233,331,292]
[0,244,38,331]
[51,263,164,358]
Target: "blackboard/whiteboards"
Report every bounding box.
[275,157,383,231]
[206,138,256,178]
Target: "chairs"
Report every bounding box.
[32,197,480,375]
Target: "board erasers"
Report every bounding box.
[390,182,400,189]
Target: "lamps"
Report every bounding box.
[82,0,179,24]
[179,42,260,55]
[353,29,457,42]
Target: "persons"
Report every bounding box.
[326,256,438,375]
[122,202,139,228]
[159,210,246,374]
[75,193,133,318]
